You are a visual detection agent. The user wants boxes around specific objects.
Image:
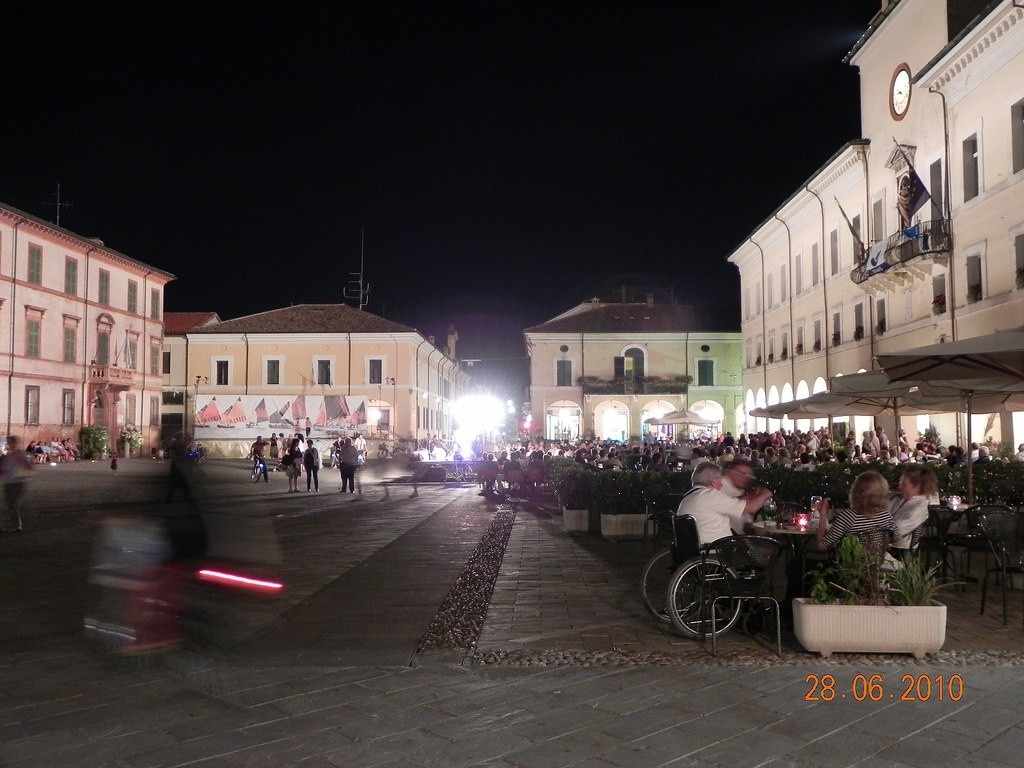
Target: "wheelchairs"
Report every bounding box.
[641,510,771,640]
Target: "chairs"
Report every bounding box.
[642,492,1024,659]
[477,464,546,498]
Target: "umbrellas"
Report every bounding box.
[829,371,1024,505]
[872,323,1024,392]
[766,391,957,461]
[644,410,706,440]
[749,398,839,456]
[650,410,720,440]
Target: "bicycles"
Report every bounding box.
[378,442,403,460]
[251,453,266,483]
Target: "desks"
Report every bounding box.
[406,460,474,483]
[749,519,827,632]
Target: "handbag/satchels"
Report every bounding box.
[282,454,294,466]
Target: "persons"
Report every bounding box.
[3,436,31,532]
[111,452,119,474]
[25,437,80,464]
[188,435,199,472]
[410,426,1024,619]
[333,435,367,493]
[270,433,320,492]
[250,436,271,483]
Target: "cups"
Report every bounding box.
[811,495,823,512]
[808,516,819,531]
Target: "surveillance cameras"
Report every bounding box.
[205,377,209,379]
[196,376,201,378]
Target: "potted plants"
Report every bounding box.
[791,535,947,659]
[80,427,110,460]
[593,470,658,542]
[546,458,595,536]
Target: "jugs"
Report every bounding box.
[764,497,777,516]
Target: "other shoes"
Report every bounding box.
[340,490,345,492]
[351,490,353,493]
[265,480,270,482]
[289,489,294,492]
[315,489,319,491]
[6,527,21,533]
[307,489,310,492]
[295,489,300,492]
